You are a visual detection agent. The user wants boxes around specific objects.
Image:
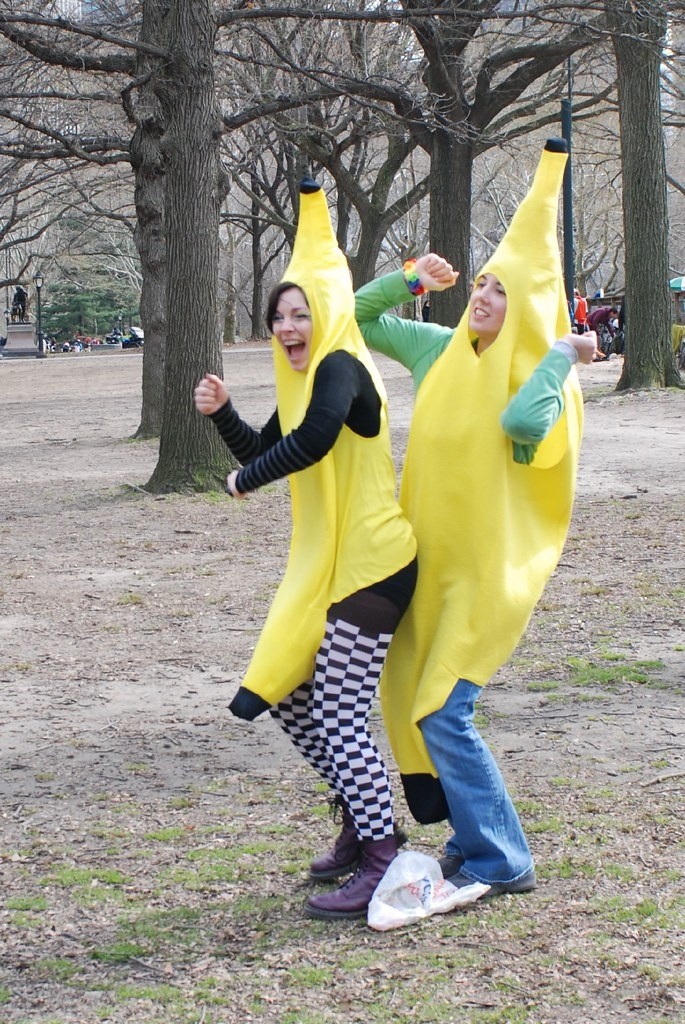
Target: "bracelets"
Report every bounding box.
[404,258,424,296]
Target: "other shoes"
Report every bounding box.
[436,858,465,880]
[444,871,536,898]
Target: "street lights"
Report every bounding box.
[32,271,50,359]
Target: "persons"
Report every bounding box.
[617,296,626,333]
[573,289,587,335]
[422,301,430,322]
[193,282,417,919]
[586,307,618,362]
[111,327,123,336]
[609,318,625,354]
[11,285,29,323]
[353,253,598,899]
[51,341,92,352]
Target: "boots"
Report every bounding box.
[307,836,398,920]
[308,797,407,878]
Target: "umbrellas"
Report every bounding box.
[670,277,685,292]
